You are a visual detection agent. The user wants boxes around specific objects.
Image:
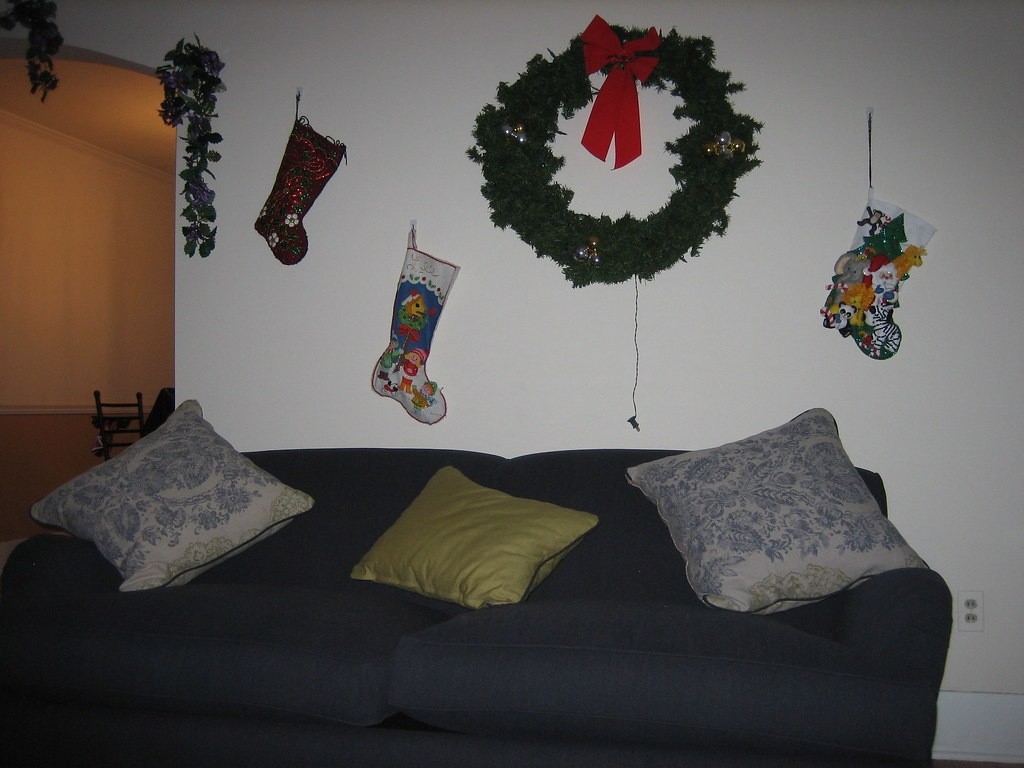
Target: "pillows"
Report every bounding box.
[30,400,319,593]
[625,408,929,616]
[350,465,600,611]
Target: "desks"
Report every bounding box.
[143,388,175,434]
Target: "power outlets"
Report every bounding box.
[956,589,985,633]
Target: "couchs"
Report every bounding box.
[0,448,953,768]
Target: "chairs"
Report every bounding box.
[93,390,145,461]
